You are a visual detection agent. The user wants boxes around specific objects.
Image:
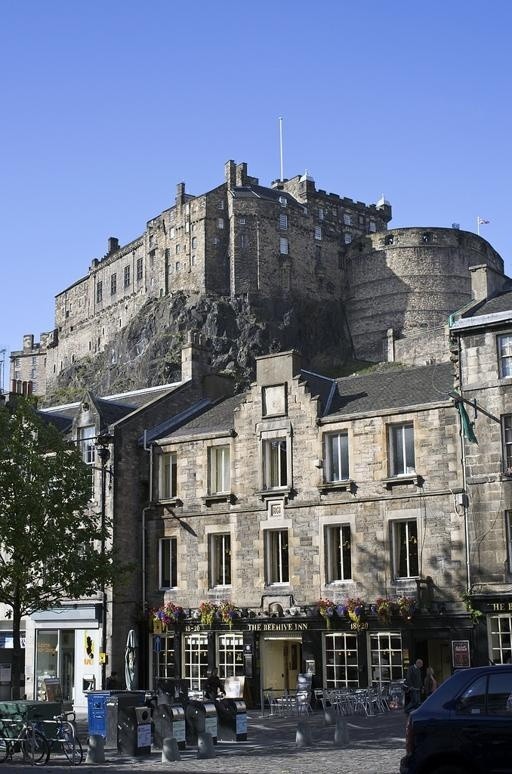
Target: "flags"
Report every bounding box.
[456,401,480,446]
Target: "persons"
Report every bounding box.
[403,658,424,715]
[204,667,226,703]
[102,672,123,688]
[420,667,437,698]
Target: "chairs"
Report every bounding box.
[264,686,390,720]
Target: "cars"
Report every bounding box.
[401,663,511,773]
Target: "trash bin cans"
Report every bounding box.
[84,690,109,743]
[0,700,61,752]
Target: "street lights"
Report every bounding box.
[95,434,114,691]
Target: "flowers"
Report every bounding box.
[307,595,417,632]
[151,600,240,630]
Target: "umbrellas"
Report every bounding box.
[124,629,141,689]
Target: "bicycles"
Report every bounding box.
[0,712,83,766]
